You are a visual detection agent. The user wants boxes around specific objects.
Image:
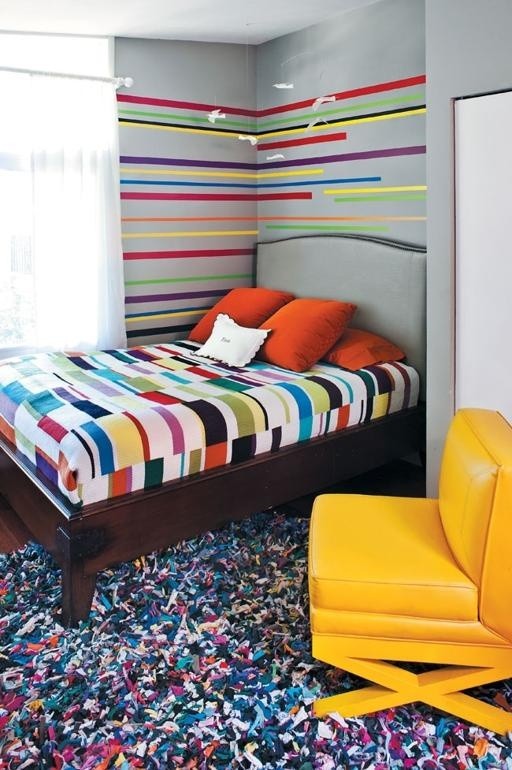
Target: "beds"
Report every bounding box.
[0,234,426,629]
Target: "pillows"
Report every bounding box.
[191,283,404,372]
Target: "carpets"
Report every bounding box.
[0,513,511,769]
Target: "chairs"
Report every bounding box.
[308,409,510,740]
